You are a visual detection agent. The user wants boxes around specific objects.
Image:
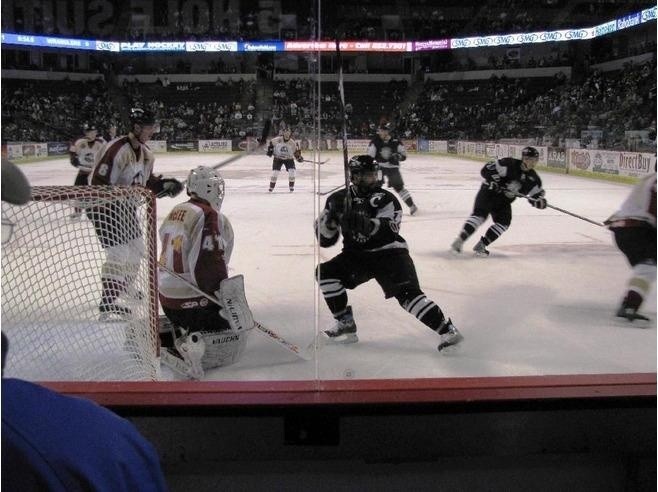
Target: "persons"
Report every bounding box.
[314,155,463,355]
[1,48,121,142]
[272,77,395,138]
[603,157,657,327]
[450,146,546,256]
[85,108,185,323]
[124,167,251,379]
[69,122,107,221]
[266,125,305,195]
[396,36,658,149]
[368,125,418,215]
[120,52,272,142]
[1,154,166,492]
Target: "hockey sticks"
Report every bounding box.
[317,160,391,196]
[334,31,352,214]
[135,118,271,208]
[264,149,330,165]
[139,252,328,361]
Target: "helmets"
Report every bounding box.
[103,118,118,131]
[186,165,226,212]
[282,127,292,136]
[374,116,396,138]
[128,105,160,128]
[521,146,540,172]
[81,119,98,131]
[349,154,385,193]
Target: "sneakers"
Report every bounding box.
[322,319,358,340]
[268,187,274,193]
[473,240,490,257]
[160,343,190,376]
[613,304,650,324]
[289,186,295,193]
[100,300,132,316]
[450,238,467,254]
[410,203,418,219]
[438,318,464,353]
[69,212,83,220]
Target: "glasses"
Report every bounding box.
[1,217,17,247]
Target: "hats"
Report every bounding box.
[0,160,32,206]
